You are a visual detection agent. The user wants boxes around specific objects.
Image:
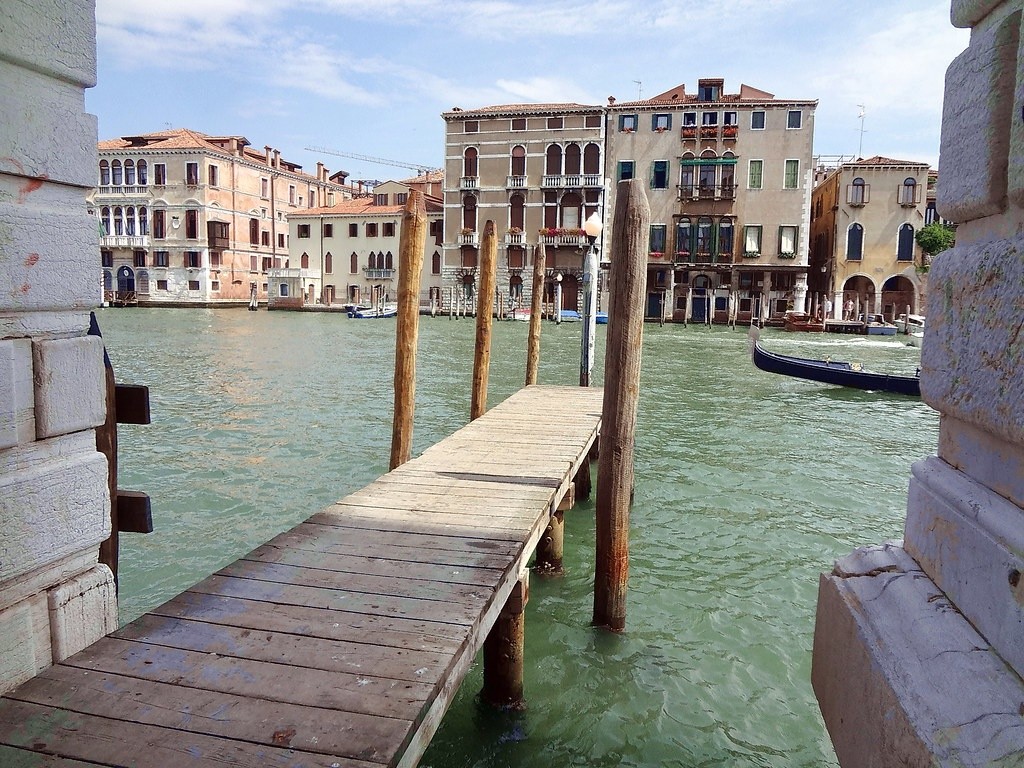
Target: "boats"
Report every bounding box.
[858,313,899,335]
[752,338,920,398]
[347,306,397,320]
[893,314,926,336]
[781,312,824,333]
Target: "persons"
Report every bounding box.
[826,297,833,319]
[843,297,854,320]
[817,304,823,321]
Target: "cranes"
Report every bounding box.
[303,145,441,188]
